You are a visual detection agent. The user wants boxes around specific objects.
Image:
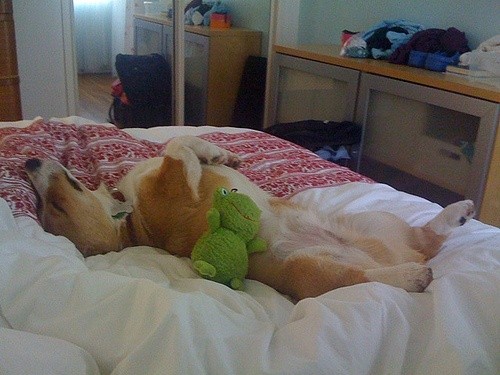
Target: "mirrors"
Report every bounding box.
[60,0,282,133]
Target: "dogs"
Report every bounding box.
[24,136,476,301]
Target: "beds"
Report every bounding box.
[0,117,500,375]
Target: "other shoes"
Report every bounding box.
[407,50,428,65]
[425,47,461,71]
[314,146,353,167]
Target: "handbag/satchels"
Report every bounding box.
[237,56,267,111]
[108,97,173,129]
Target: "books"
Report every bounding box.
[445,65,493,77]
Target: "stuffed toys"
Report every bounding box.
[195,189,267,290]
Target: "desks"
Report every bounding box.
[133,13,263,127]
[268,41,500,223]
